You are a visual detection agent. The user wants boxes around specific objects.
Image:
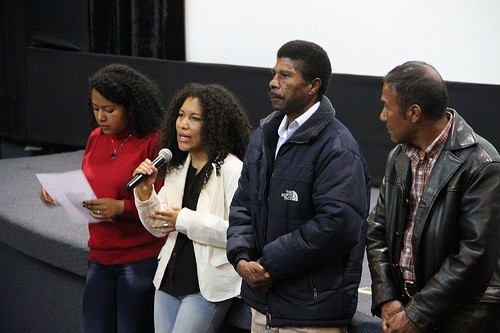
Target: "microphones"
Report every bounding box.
[126,148,172,192]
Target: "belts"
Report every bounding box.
[401,281,417,300]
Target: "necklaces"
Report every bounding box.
[108,130,134,160]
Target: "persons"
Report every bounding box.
[132,82,253,333]
[224,39,369,333]
[39,63,173,332]
[363,60,499,333]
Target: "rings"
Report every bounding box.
[164,223,169,227]
[98,211,100,214]
